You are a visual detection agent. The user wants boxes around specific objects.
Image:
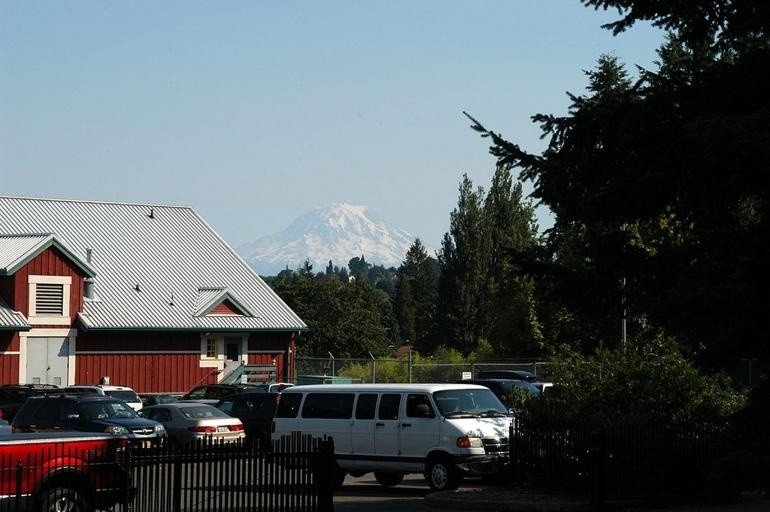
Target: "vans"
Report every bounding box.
[271,382,525,491]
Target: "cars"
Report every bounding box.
[0,382,297,512]
[457,370,569,409]
